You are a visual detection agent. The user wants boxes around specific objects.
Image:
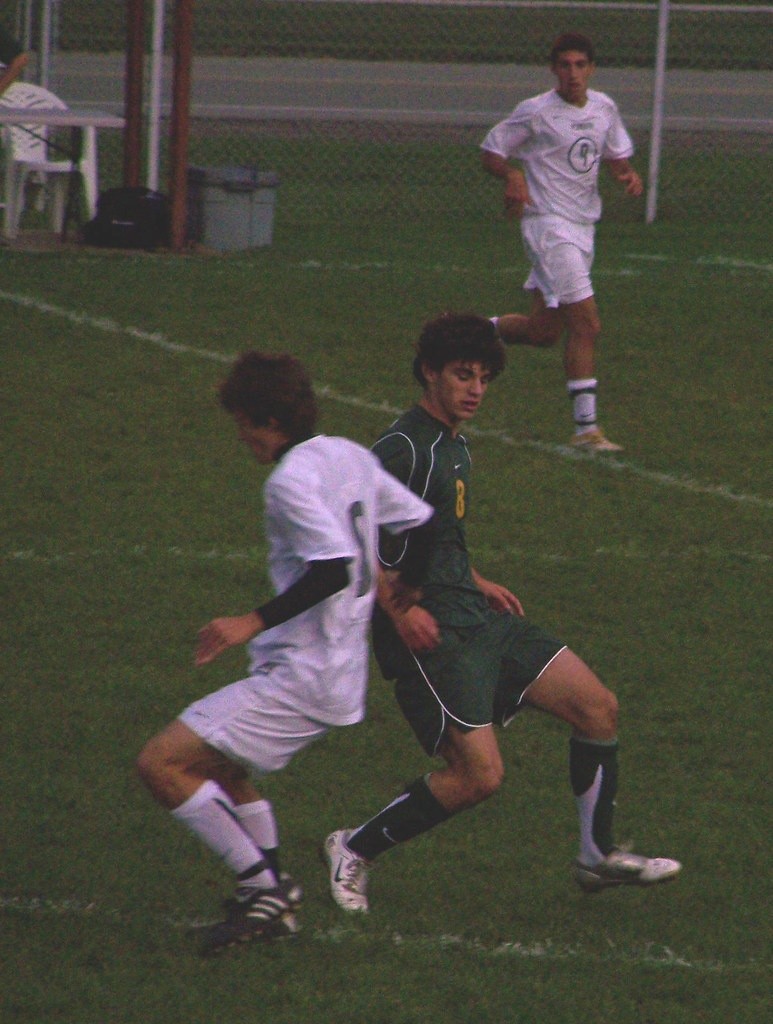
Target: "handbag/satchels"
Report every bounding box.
[95,184,172,242]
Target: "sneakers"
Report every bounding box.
[571,428,625,451]
[206,886,294,954]
[278,874,303,909]
[577,851,682,891]
[323,829,370,914]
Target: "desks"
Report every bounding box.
[0,105,128,241]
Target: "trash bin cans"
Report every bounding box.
[186,162,281,255]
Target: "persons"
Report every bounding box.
[136,346,439,956]
[323,313,682,915]
[476,29,643,453]
[0,27,29,211]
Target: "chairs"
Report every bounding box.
[0,80,78,235]
